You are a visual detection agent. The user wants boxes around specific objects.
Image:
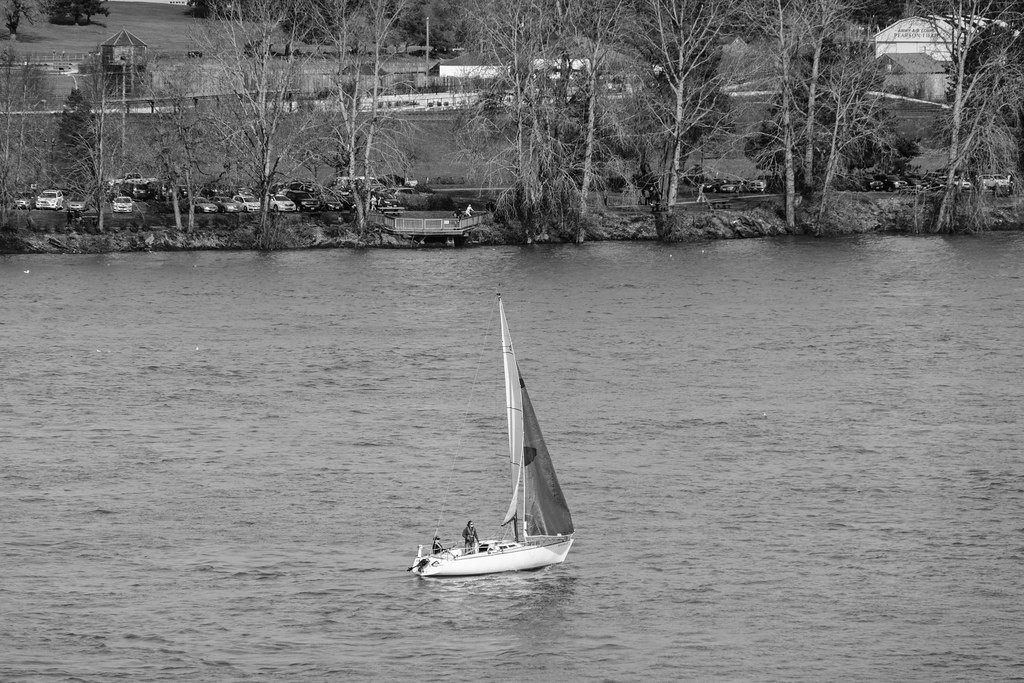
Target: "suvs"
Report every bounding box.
[35,190,64,210]
[286,191,320,212]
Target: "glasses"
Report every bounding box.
[436,538,440,540]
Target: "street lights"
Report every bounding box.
[67,74,78,111]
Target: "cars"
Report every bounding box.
[208,196,243,213]
[387,188,416,206]
[749,174,773,192]
[834,175,883,192]
[939,176,972,189]
[370,190,401,211]
[233,195,261,213]
[697,178,746,193]
[259,194,296,213]
[873,173,908,192]
[180,197,218,213]
[112,196,135,213]
[12,192,36,210]
[188,50,202,58]
[67,197,89,211]
[319,195,343,212]
[978,174,1014,190]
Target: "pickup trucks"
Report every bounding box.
[108,172,157,186]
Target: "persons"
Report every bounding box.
[453,203,475,230]
[637,187,662,205]
[697,184,705,202]
[604,189,609,206]
[370,194,377,212]
[66,209,83,227]
[462,521,479,554]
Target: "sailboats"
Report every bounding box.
[407,292,576,577]
